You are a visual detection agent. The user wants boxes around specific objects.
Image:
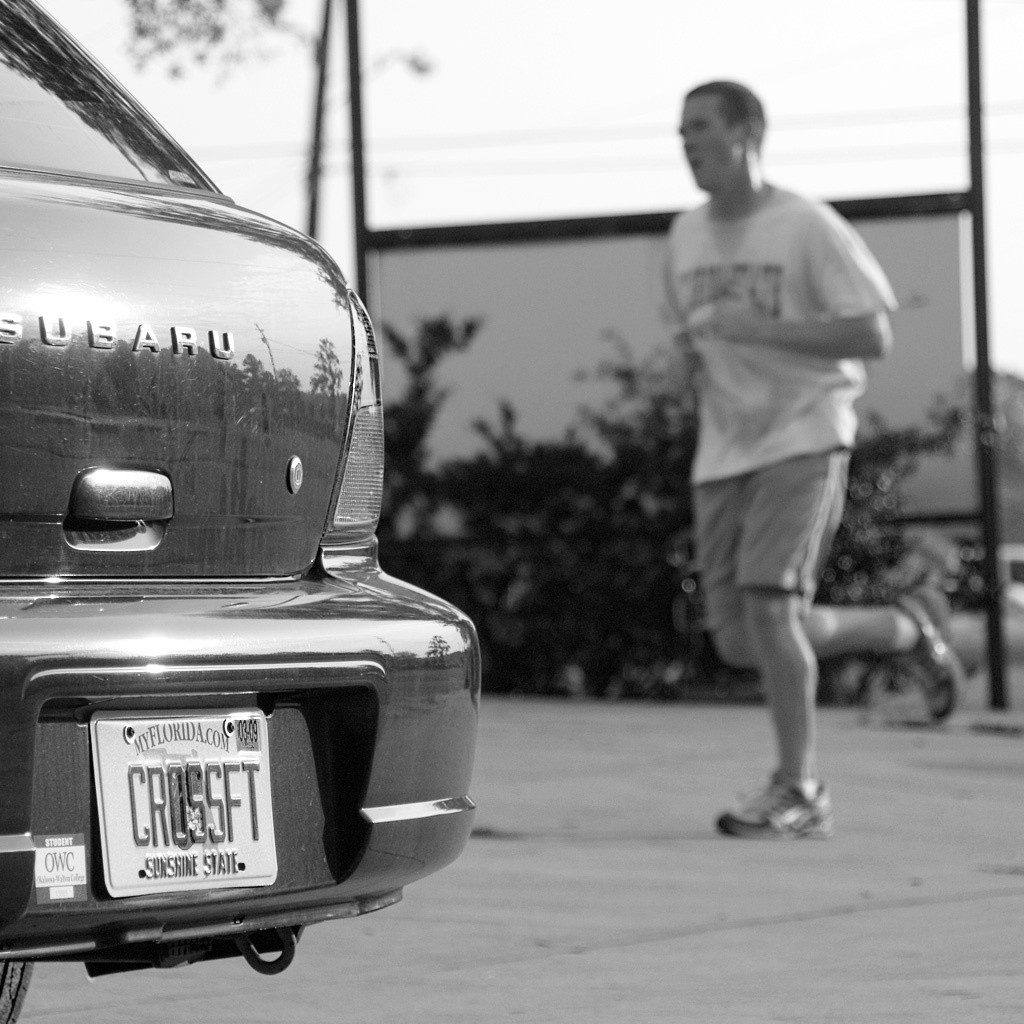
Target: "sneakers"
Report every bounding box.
[891,595,961,719]
[719,774,835,839]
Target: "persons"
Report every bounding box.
[663,82,970,842]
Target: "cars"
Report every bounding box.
[0,0,483,1024]
